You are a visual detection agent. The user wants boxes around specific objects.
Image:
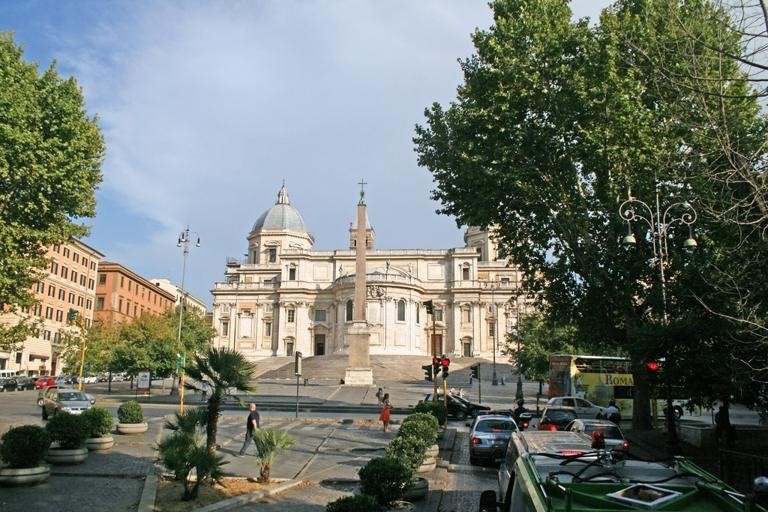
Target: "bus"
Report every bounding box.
[547,355,687,418]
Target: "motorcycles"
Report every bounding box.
[507,408,533,429]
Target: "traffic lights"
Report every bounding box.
[423,300,433,314]
[645,360,658,381]
[422,364,432,382]
[69,308,79,321]
[470,365,478,379]
[441,358,450,379]
[433,358,441,377]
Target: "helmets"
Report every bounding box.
[609,399,615,406]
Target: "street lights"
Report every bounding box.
[176,224,202,343]
[490,281,499,386]
[618,179,699,459]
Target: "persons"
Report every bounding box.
[76,374,80,390]
[376,387,384,407]
[233,403,264,457]
[379,393,393,432]
[202,380,208,396]
[715,406,725,423]
[601,400,618,420]
[200,381,211,401]
[70,393,77,400]
[612,364,633,374]
[436,386,463,397]
[729,425,736,447]
[71,376,77,389]
[514,399,525,420]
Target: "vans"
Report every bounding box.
[498,430,613,499]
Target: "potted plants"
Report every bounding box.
[80,407,114,450]
[403,412,439,457]
[414,401,448,438]
[385,434,428,500]
[45,411,88,464]
[116,400,148,433]
[401,420,436,472]
[0,425,51,484]
[358,455,417,512]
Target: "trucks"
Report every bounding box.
[479,451,767,512]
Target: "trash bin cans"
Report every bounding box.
[304,379,308,386]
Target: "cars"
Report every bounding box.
[547,395,606,418]
[567,419,628,454]
[37,385,96,406]
[42,389,92,419]
[0,368,130,391]
[539,408,577,431]
[452,392,492,417]
[424,394,467,420]
[470,415,517,460]
